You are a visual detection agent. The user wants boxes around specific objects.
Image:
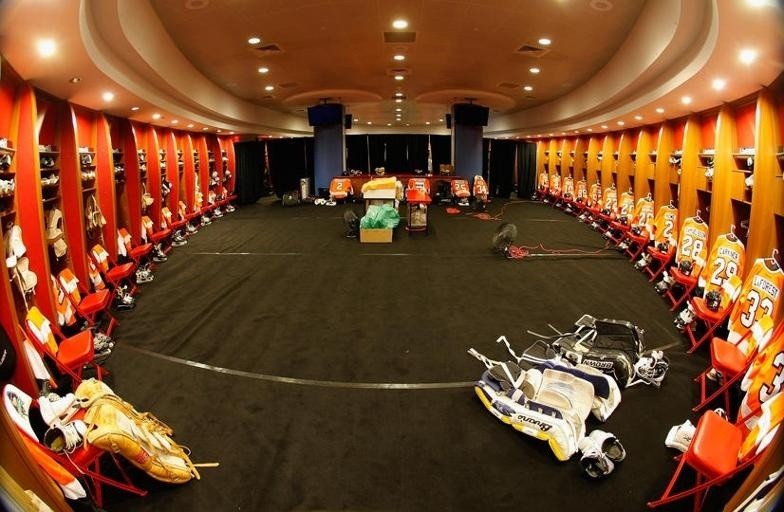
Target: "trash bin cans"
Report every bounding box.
[299,178,310,201]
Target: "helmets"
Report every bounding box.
[602,209,610,216]
[679,261,693,276]
[632,227,640,235]
[704,291,720,312]
[619,216,628,225]
[541,185,592,207]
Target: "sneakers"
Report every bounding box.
[706,368,723,385]
[42,418,88,474]
[589,429,626,463]
[676,311,697,332]
[148,205,234,262]
[673,308,690,324]
[578,436,614,479]
[37,393,75,425]
[638,351,671,372]
[636,364,666,383]
[91,266,154,352]
[616,243,678,294]
[580,212,615,241]
